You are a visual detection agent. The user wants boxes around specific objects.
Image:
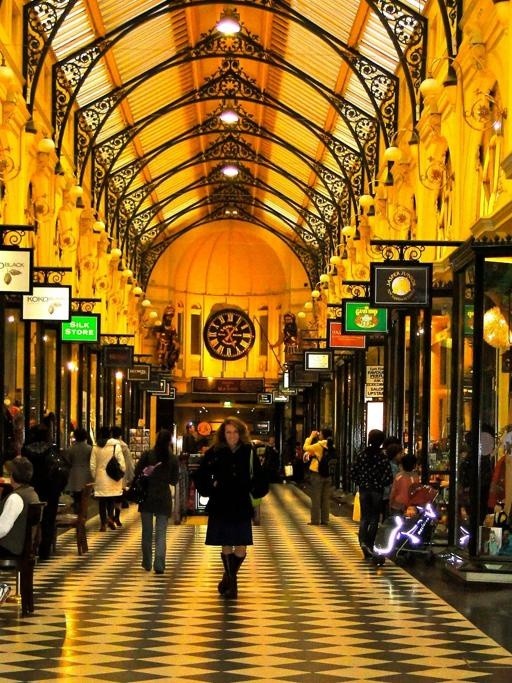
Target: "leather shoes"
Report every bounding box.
[100,518,121,532]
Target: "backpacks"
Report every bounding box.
[317,442,338,476]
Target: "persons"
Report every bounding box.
[182,422,198,454]
[192,417,269,599]
[133,428,178,575]
[303,427,335,525]
[349,427,451,560]
[1,411,136,605]
[262,435,275,466]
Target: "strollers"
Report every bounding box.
[368,483,448,567]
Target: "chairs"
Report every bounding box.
[0,502,47,617]
[53,484,96,556]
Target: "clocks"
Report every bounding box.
[203,309,255,360]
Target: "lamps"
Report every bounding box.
[216,10,241,178]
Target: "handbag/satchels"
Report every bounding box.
[38,451,68,492]
[106,456,124,481]
[126,475,168,505]
[249,449,262,508]
[353,492,361,522]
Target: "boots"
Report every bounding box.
[218,552,246,599]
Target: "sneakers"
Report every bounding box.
[142,560,164,574]
[360,543,373,559]
[308,522,329,525]
[0,584,11,602]
